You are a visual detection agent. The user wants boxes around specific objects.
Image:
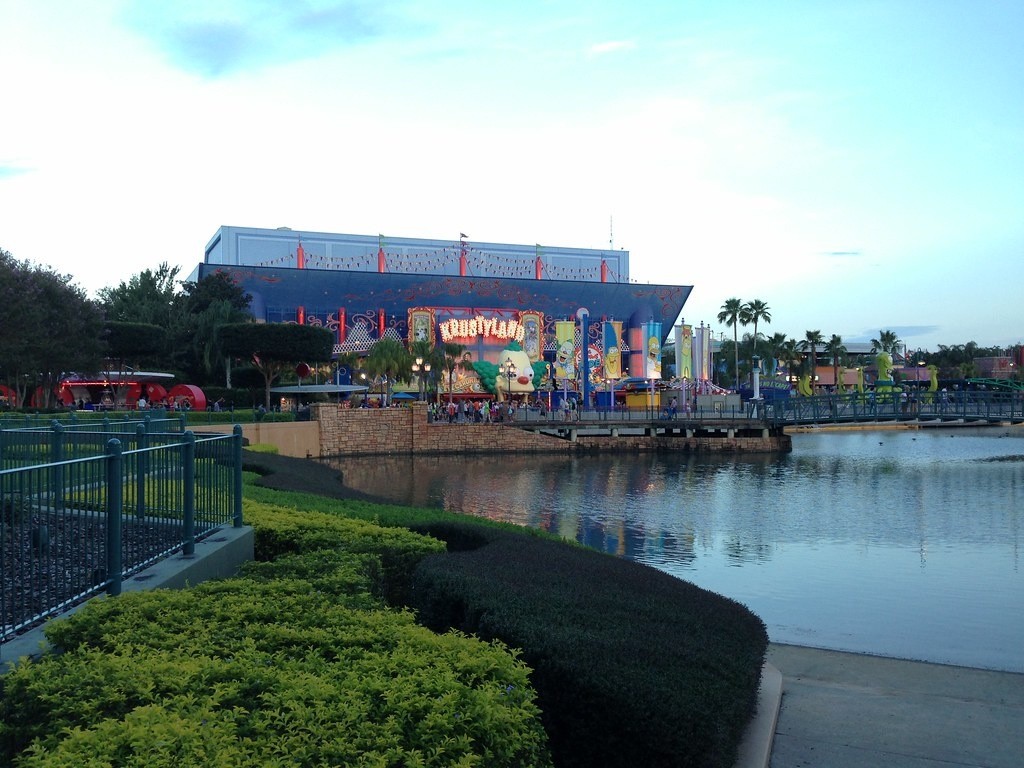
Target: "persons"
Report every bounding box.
[2,398,10,413]
[207,398,212,412]
[214,402,220,413]
[298,402,303,412]
[153,396,193,412]
[258,404,263,413]
[558,392,583,422]
[428,398,514,423]
[537,398,549,422]
[868,387,874,413]
[359,398,400,408]
[139,397,146,411]
[939,384,949,413]
[59,398,93,410]
[900,384,918,413]
[685,399,692,421]
[670,396,678,421]
[145,400,151,408]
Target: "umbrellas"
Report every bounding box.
[390,391,415,398]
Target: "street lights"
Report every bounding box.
[499,356,516,403]
[916,346,925,415]
[411,355,431,400]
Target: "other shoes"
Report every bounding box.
[510,421,513,423]
